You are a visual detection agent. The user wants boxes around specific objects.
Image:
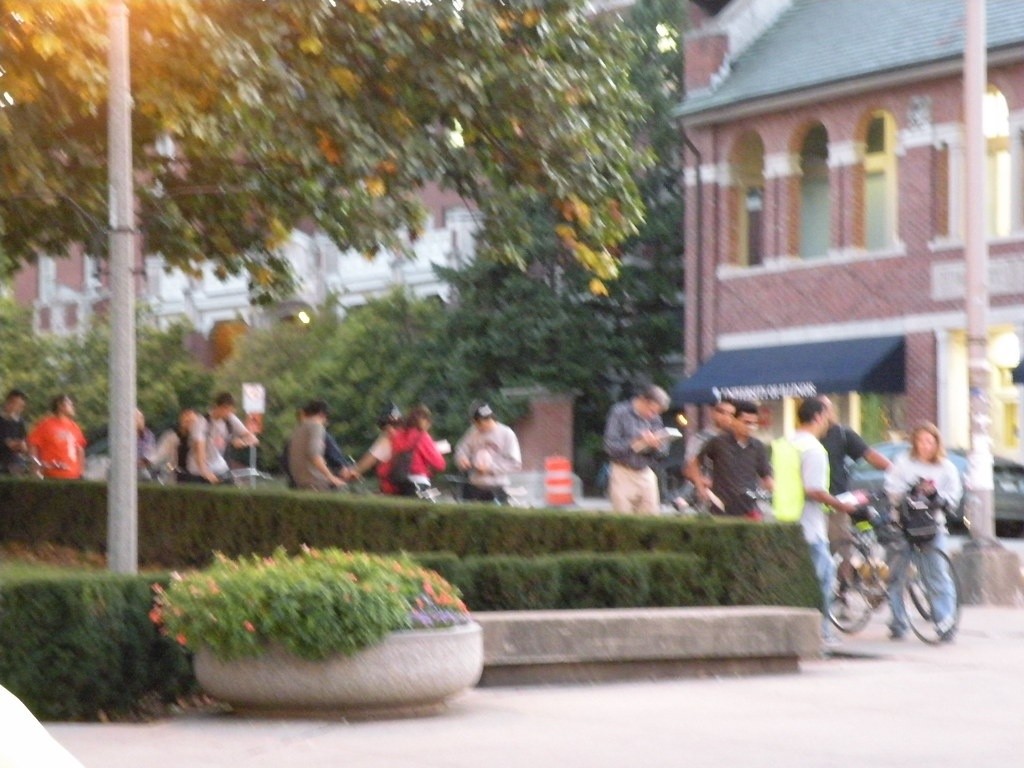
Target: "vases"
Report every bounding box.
[193,618,484,723]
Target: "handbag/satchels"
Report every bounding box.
[387,450,411,485]
[899,491,938,544]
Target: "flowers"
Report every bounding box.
[148,544,472,666]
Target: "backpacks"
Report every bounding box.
[177,412,231,469]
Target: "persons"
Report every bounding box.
[770,398,856,656]
[281,406,356,482]
[681,399,736,512]
[690,400,771,522]
[0,390,32,475]
[286,400,350,492]
[884,418,964,639]
[603,383,673,513]
[338,407,400,493]
[391,402,446,502]
[453,405,522,502]
[810,394,894,622]
[186,391,259,482]
[26,393,89,481]
[138,407,199,483]
[136,406,155,479]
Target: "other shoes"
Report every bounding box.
[889,622,906,639]
[940,630,951,640]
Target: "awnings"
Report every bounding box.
[668,333,906,402]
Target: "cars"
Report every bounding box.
[846,442,1023,540]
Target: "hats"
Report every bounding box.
[471,404,493,417]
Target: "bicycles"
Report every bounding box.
[3,430,778,521]
[827,486,963,647]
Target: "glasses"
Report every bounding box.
[737,420,758,424]
